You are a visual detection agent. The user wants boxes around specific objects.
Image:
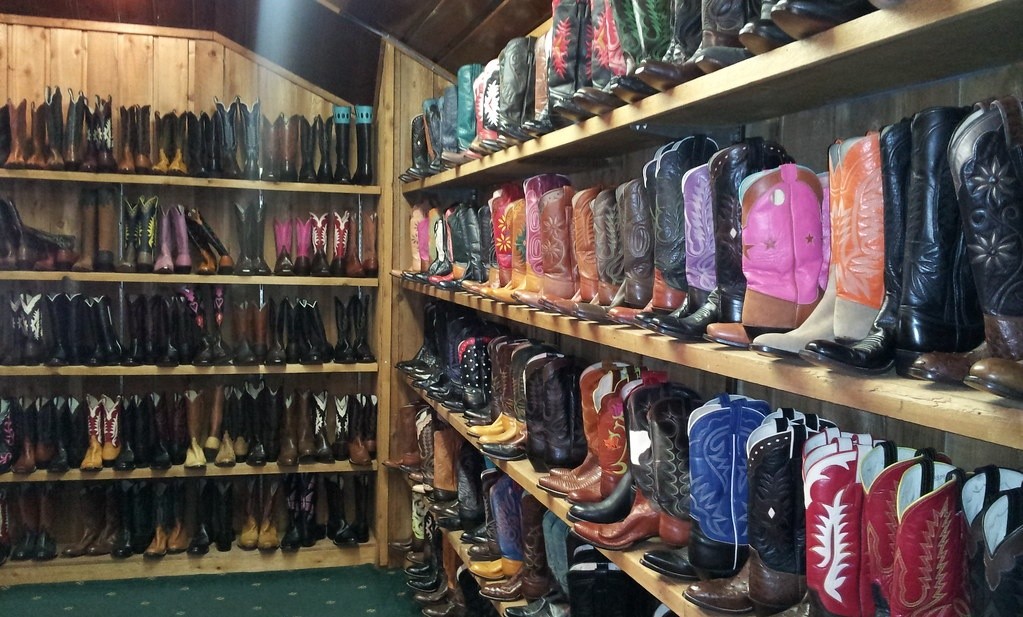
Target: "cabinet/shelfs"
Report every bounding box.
[0,168,386,584]
[395,0,1023,617]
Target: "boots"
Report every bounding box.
[0,382,376,473]
[0,474,371,566]
[398,0,867,185]
[4,284,375,367]
[381,96,1023,617]
[0,86,376,185]
[0,193,377,277]
